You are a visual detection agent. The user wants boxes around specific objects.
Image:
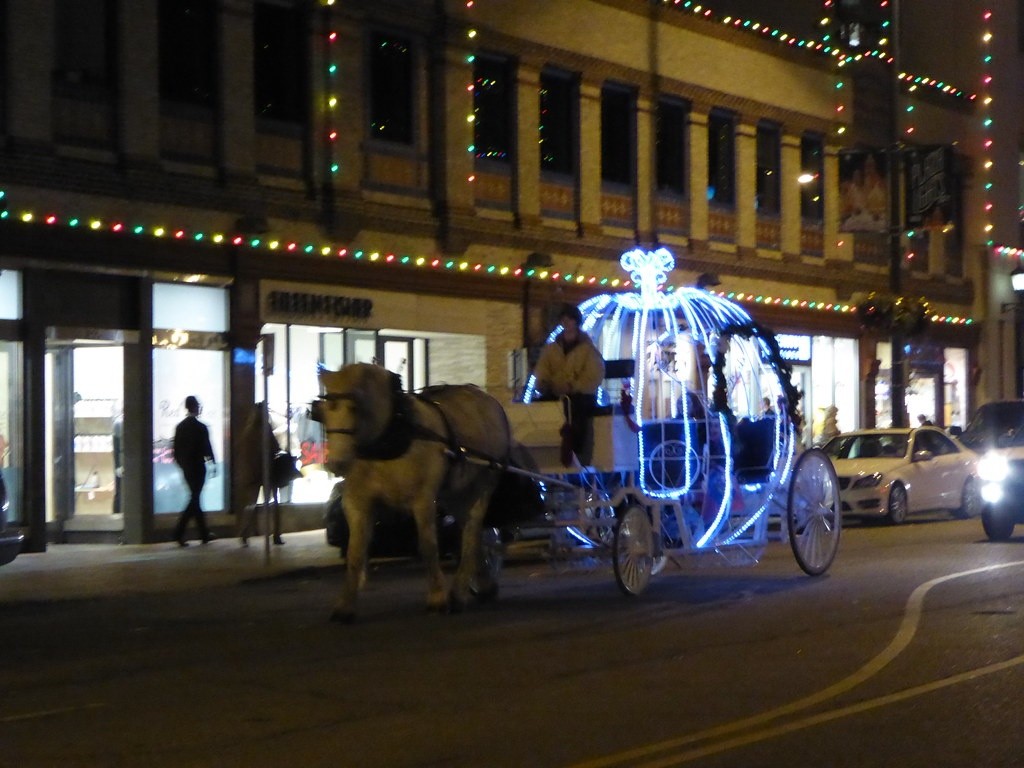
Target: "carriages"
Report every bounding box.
[310,244,842,630]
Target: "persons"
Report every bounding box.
[239,402,285,546]
[173,396,214,547]
[824,406,840,438]
[918,415,932,427]
[763,398,774,415]
[535,302,605,466]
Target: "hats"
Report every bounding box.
[561,304,582,323]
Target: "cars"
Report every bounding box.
[322,467,553,571]
[949,403,1024,456]
[820,428,978,527]
[975,426,1024,543]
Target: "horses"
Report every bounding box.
[314,362,512,626]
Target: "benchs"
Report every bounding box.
[544,418,715,504]
[698,415,778,480]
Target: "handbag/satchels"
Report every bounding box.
[271,450,303,488]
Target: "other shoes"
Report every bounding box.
[203,533,217,543]
[176,538,189,547]
